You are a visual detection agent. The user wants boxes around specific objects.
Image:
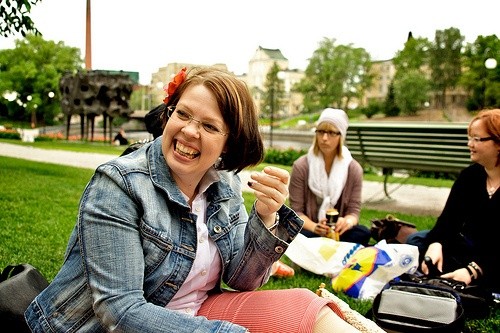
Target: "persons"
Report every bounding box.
[24,69,361,332]
[287,108,371,247]
[405,109,500,294]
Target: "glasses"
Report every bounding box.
[315,129,342,136]
[166,105,231,137]
[466,135,493,142]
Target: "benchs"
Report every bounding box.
[344,123,477,200]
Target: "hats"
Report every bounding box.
[313,106,349,141]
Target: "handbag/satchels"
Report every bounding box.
[371,263,493,333]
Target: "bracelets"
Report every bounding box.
[468,263,482,279]
[465,266,474,283]
[268,213,280,232]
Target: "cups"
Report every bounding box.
[326,209,340,231]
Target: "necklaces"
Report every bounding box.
[487,177,500,198]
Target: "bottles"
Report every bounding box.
[423,256,444,279]
